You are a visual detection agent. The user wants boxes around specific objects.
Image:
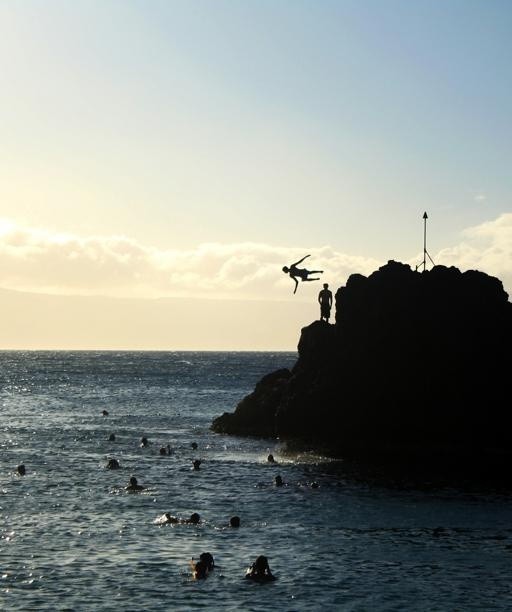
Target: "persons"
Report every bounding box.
[281,253,324,295]
[17,463,28,476]
[318,283,333,324]
[189,551,223,573]
[228,515,242,528]
[101,407,321,494]
[243,555,277,583]
[163,512,203,525]
[192,562,209,581]
